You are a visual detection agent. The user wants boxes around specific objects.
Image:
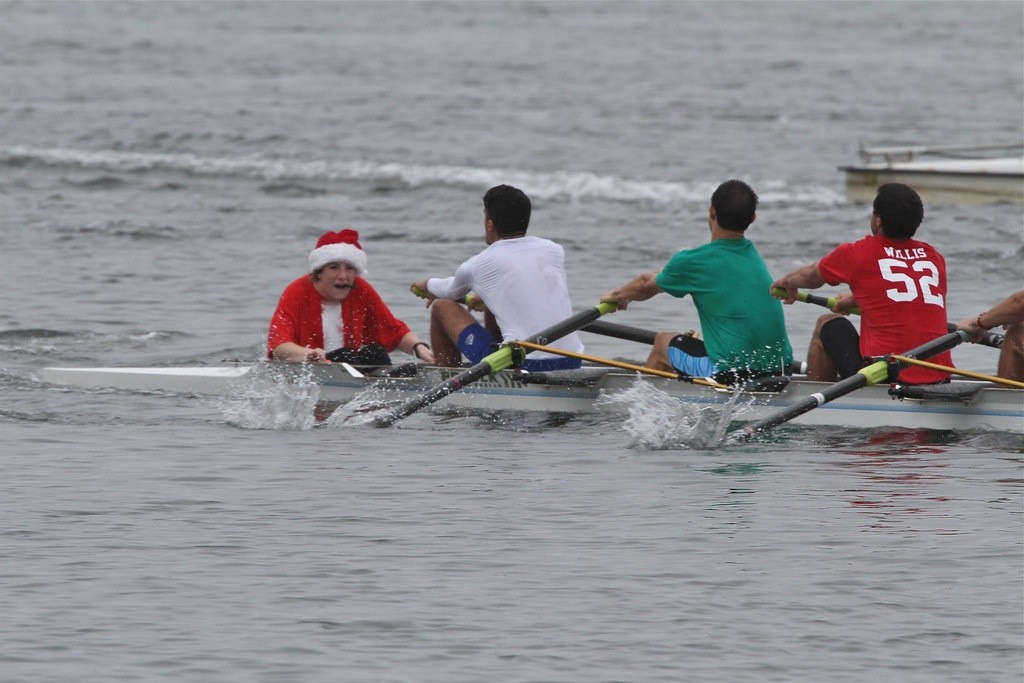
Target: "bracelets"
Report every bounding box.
[412,342,430,359]
[977,312,991,330]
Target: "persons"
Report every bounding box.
[958,289,1024,388]
[600,178,794,392]
[266,229,434,365]
[412,184,586,371]
[770,183,957,388]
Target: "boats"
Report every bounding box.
[32,359,1024,436]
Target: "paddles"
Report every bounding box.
[373,283,1024,443]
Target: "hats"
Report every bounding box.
[310,230,366,275]
